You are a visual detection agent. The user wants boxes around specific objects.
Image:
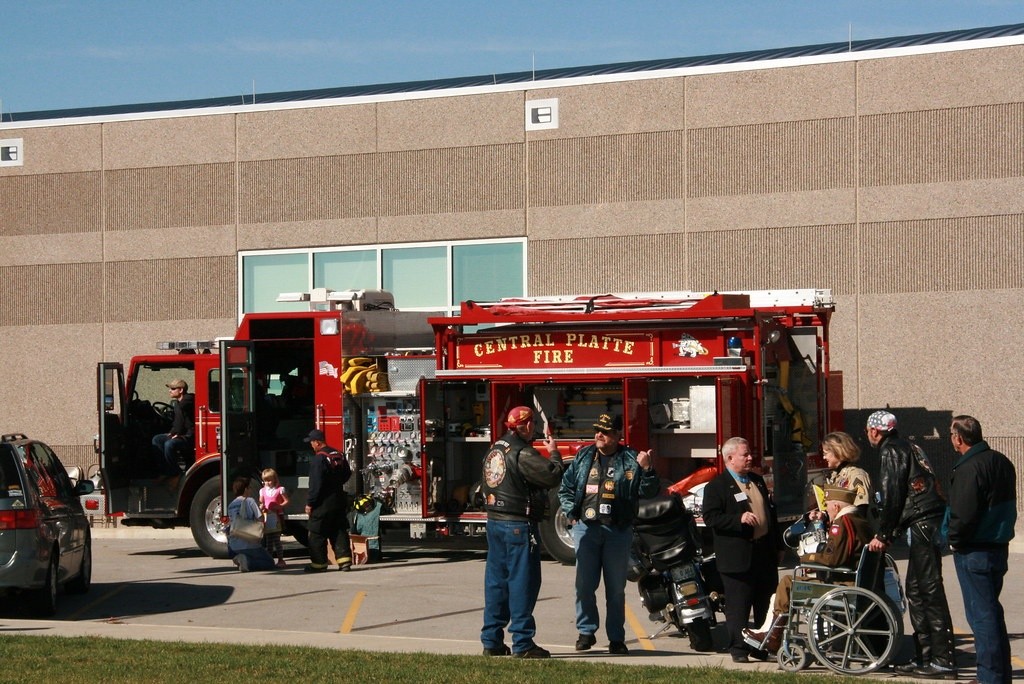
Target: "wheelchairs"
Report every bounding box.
[742,542,904,676]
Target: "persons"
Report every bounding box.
[481,407,565,659]
[558,412,659,657]
[228,476,275,573]
[867,410,959,680]
[703,436,781,664]
[742,431,891,670]
[152,380,196,493]
[303,429,352,573]
[941,415,1017,684]
[257,468,291,569]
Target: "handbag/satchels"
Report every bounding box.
[230,497,264,542]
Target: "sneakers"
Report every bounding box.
[575,634,596,650]
[512,645,551,658]
[483,644,511,655]
[609,642,628,654]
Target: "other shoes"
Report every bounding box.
[339,566,351,571]
[277,560,287,568]
[732,655,749,662]
[233,553,249,572]
[305,565,327,572]
[763,654,777,662]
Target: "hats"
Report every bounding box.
[823,483,856,505]
[593,413,623,431]
[867,411,897,431]
[303,430,325,442]
[165,380,188,391]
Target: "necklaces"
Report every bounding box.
[729,469,750,483]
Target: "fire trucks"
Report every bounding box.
[90,287,837,567]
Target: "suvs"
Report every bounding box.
[0,430,96,614]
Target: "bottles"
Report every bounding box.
[814,518,826,543]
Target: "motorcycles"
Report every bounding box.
[630,483,724,652]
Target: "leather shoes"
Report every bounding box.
[912,664,958,680]
[894,663,919,676]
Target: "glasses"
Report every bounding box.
[593,426,616,435]
[530,420,539,424]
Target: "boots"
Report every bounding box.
[742,615,789,656]
